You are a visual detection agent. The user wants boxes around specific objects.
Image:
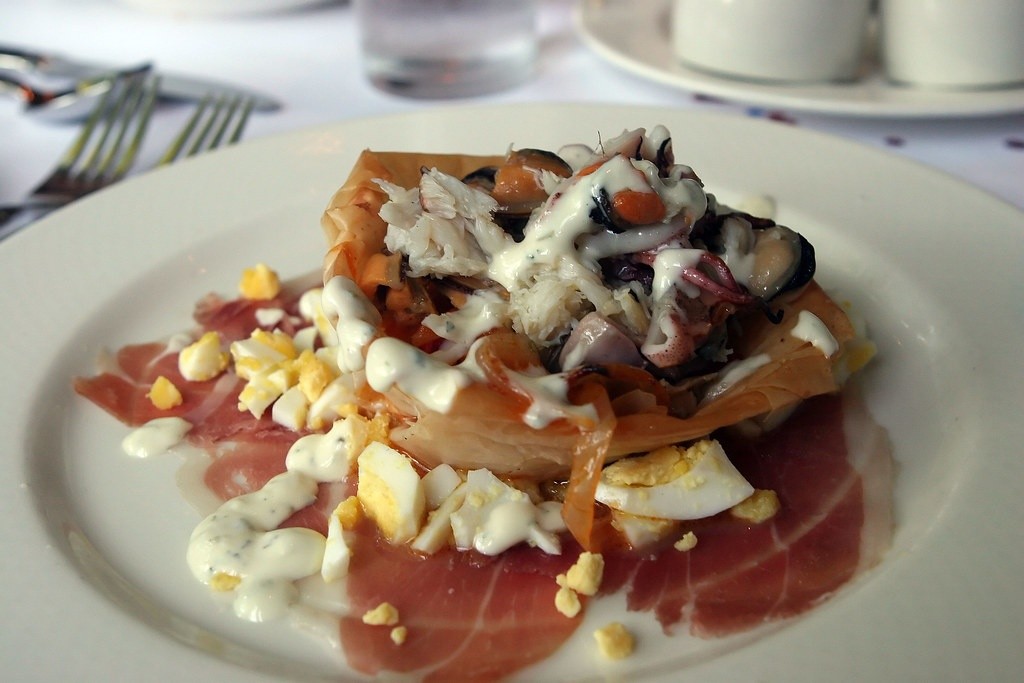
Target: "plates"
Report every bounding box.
[574,0,1024,116]
[0,105,1024,683]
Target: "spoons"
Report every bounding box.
[0,69,109,122]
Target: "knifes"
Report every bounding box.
[0,46,280,112]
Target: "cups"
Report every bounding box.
[670,0,871,82]
[356,0,538,98]
[882,0,1024,88]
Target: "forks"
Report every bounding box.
[156,95,258,166]
[0,86,158,239]
